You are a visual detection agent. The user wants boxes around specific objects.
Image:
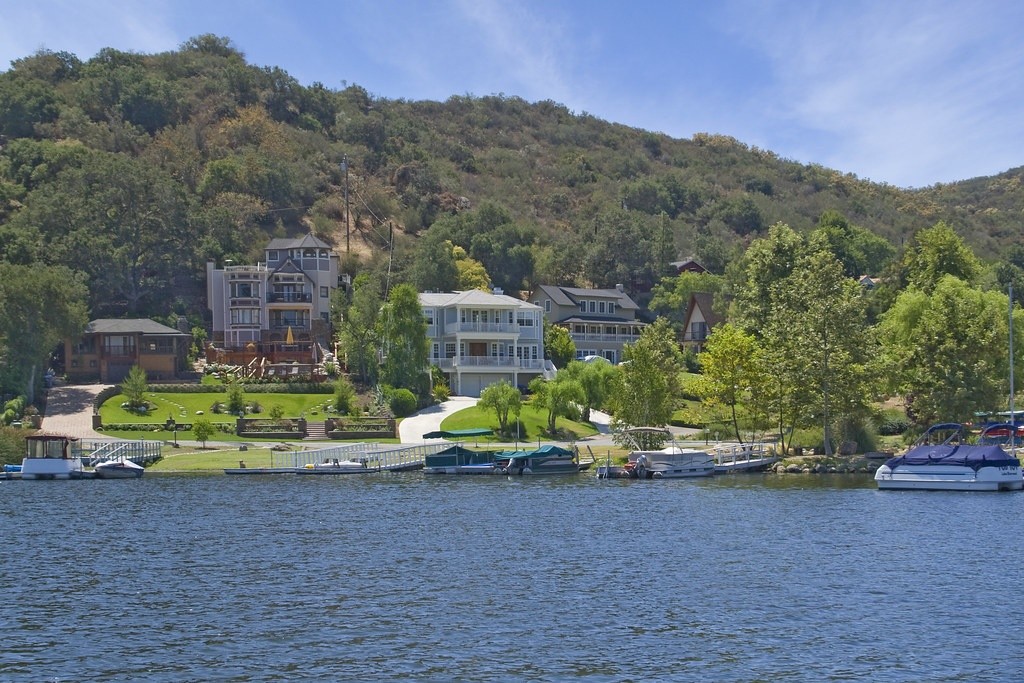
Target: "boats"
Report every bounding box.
[293,460,378,474]
[490,443,595,476]
[871,421,1024,493]
[94,459,144,478]
[624,425,716,479]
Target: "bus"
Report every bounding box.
[960,411,1024,439]
